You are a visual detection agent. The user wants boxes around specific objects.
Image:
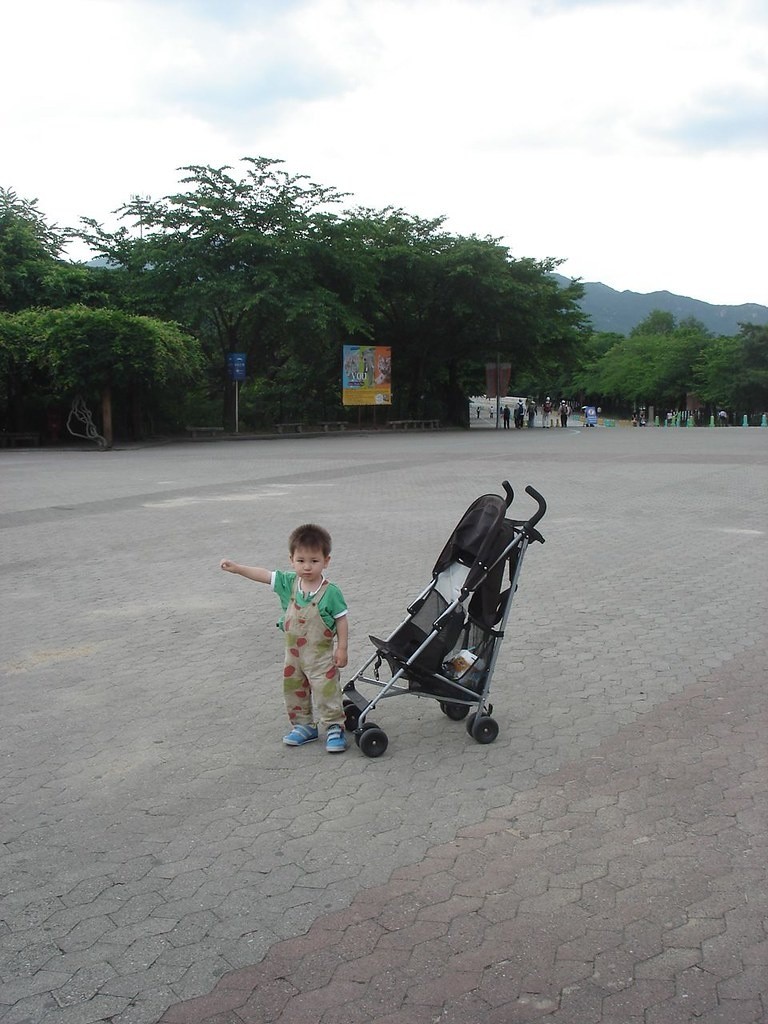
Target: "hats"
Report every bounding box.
[531,401,535,403]
[546,397,550,401]
[561,400,566,404]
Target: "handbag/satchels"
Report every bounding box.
[641,419,645,424]
[631,419,636,423]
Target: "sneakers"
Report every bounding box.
[325,723,347,752]
[282,721,319,745]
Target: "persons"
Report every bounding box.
[222,523,349,751]
[476,397,731,429]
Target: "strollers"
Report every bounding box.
[341,481,548,758]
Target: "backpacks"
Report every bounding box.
[516,402,525,413]
[561,405,567,412]
[544,402,551,411]
